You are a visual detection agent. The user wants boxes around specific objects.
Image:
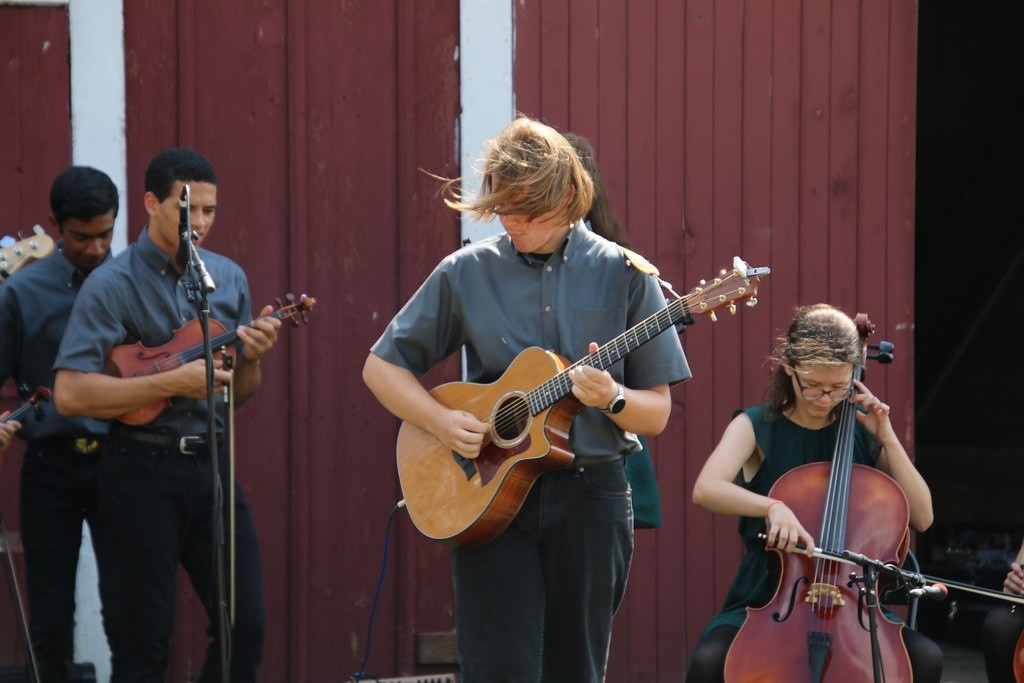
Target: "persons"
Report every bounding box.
[983,538,1024,683]
[363,117,693,683]
[687,304,943,683]
[54,147,282,683]
[0,165,120,683]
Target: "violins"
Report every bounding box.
[0,383,53,431]
[103,291,316,425]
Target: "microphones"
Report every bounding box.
[176,187,193,268]
[908,584,948,601]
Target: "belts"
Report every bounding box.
[37,436,105,455]
[115,426,224,455]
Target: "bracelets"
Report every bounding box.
[766,500,784,517]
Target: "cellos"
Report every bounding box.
[722,311,917,683]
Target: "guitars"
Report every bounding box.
[394,254,778,543]
[0,223,56,282]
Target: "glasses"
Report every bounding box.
[793,364,854,401]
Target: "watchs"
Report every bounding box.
[595,384,627,414]
